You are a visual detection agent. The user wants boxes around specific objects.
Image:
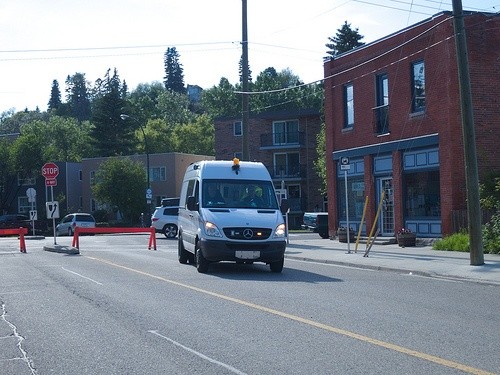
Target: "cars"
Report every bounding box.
[55,213,95,236]
[0,214,33,235]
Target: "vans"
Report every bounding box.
[161,198,179,206]
[177,161,288,274]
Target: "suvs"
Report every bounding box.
[151,205,180,237]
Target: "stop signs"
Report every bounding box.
[42,163,59,179]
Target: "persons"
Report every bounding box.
[205,186,226,207]
[242,184,266,207]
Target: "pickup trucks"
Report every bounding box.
[301,212,329,238]
